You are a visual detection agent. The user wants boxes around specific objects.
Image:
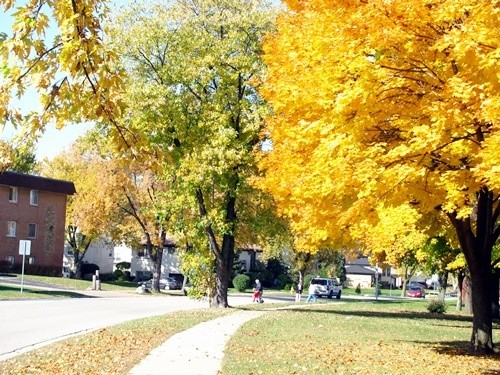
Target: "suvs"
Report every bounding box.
[308,277,342,299]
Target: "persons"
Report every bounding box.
[253,279,263,303]
[295,282,303,302]
[306,281,320,303]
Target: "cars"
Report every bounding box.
[137,276,181,290]
[406,286,425,298]
